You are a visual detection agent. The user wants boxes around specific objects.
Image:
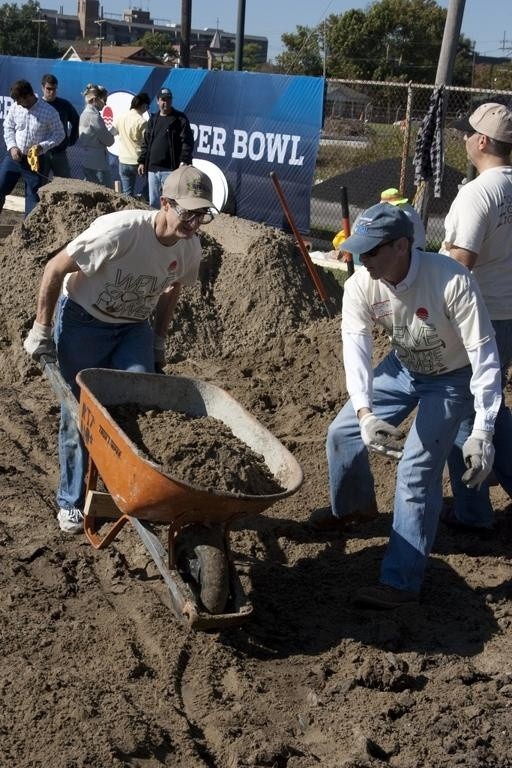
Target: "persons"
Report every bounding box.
[378,186,426,252]
[22,165,219,536]
[307,201,502,611]
[439,103,512,538]
[1,73,194,211]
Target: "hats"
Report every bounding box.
[337,202,414,254]
[332,229,352,259]
[454,103,511,143]
[162,165,220,215]
[157,88,172,98]
[379,188,409,207]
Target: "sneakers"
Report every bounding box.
[352,585,420,608]
[309,506,380,531]
[440,505,495,542]
[56,507,84,535]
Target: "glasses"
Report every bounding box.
[174,208,214,224]
[368,234,406,257]
[46,87,56,90]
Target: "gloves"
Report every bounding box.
[358,412,405,460]
[23,320,56,362]
[152,331,168,370]
[27,145,40,172]
[461,430,495,492]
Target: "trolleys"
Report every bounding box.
[22,326,308,632]
